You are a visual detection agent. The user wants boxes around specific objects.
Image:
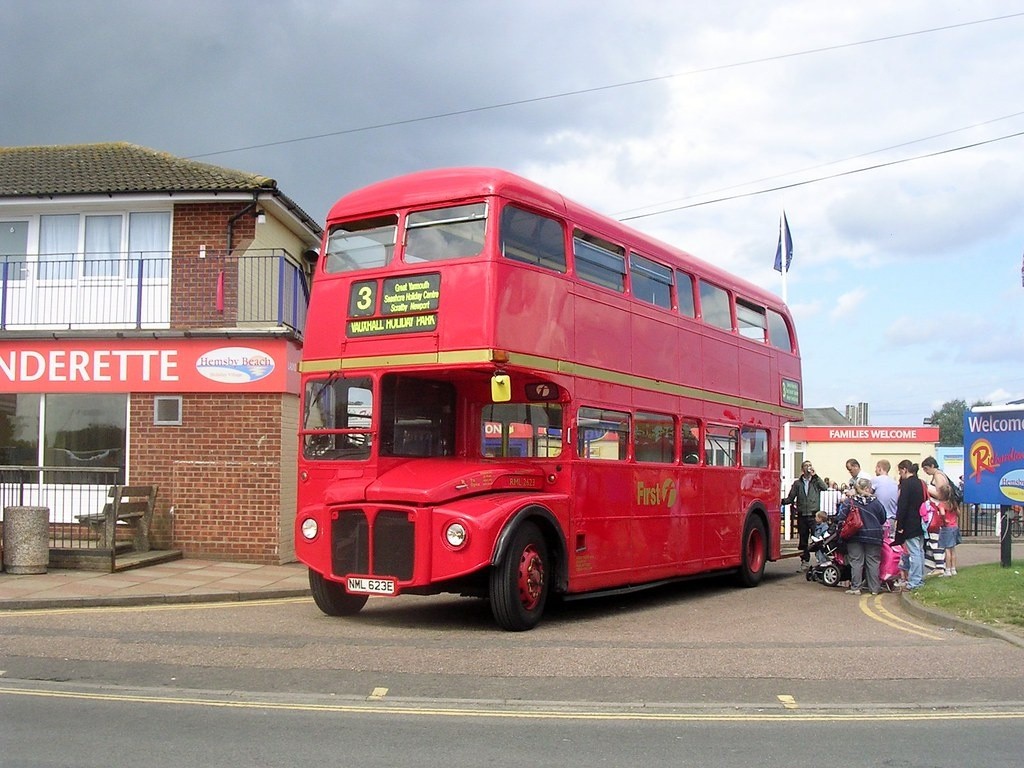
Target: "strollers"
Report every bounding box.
[805,523,863,587]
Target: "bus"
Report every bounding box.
[294,163,805,634]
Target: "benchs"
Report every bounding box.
[72,484,160,559]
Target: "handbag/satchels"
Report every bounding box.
[840,500,863,539]
[920,479,939,533]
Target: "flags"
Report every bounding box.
[773,210,793,274]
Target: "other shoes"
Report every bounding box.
[927,569,945,575]
[893,579,906,586]
[951,571,957,575]
[845,589,862,595]
[938,572,952,577]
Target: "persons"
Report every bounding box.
[834,479,886,596]
[844,459,899,537]
[935,484,963,579]
[922,456,951,575]
[810,511,831,574]
[890,460,929,591]
[845,459,874,499]
[957,475,985,516]
[894,542,909,586]
[824,477,847,490]
[780,461,828,575]
